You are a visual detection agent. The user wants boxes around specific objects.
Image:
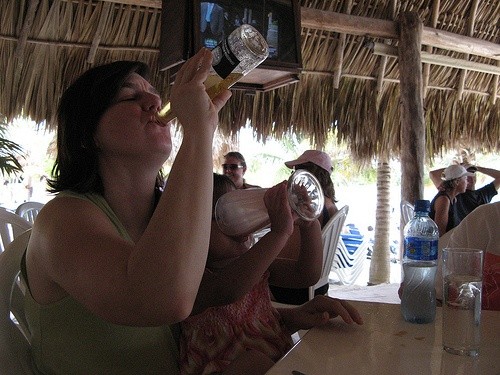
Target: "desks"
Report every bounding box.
[265,300,500,375]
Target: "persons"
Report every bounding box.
[428,162,500,220]
[269,149,339,305]
[200,2,277,57]
[176,172,323,375]
[397,200,500,310]
[221,152,261,189]
[20,47,362,375]
[429,165,475,237]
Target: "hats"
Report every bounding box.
[285,150,334,176]
[463,163,478,172]
[440,165,475,181]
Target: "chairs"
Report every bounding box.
[309,205,367,299]
[0,201,45,375]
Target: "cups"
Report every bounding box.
[215,169,324,237]
[442,247,483,357]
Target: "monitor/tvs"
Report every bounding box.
[162,0,303,95]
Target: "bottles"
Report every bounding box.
[401,200,439,324]
[154,24,269,126]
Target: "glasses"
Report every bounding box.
[222,164,244,170]
